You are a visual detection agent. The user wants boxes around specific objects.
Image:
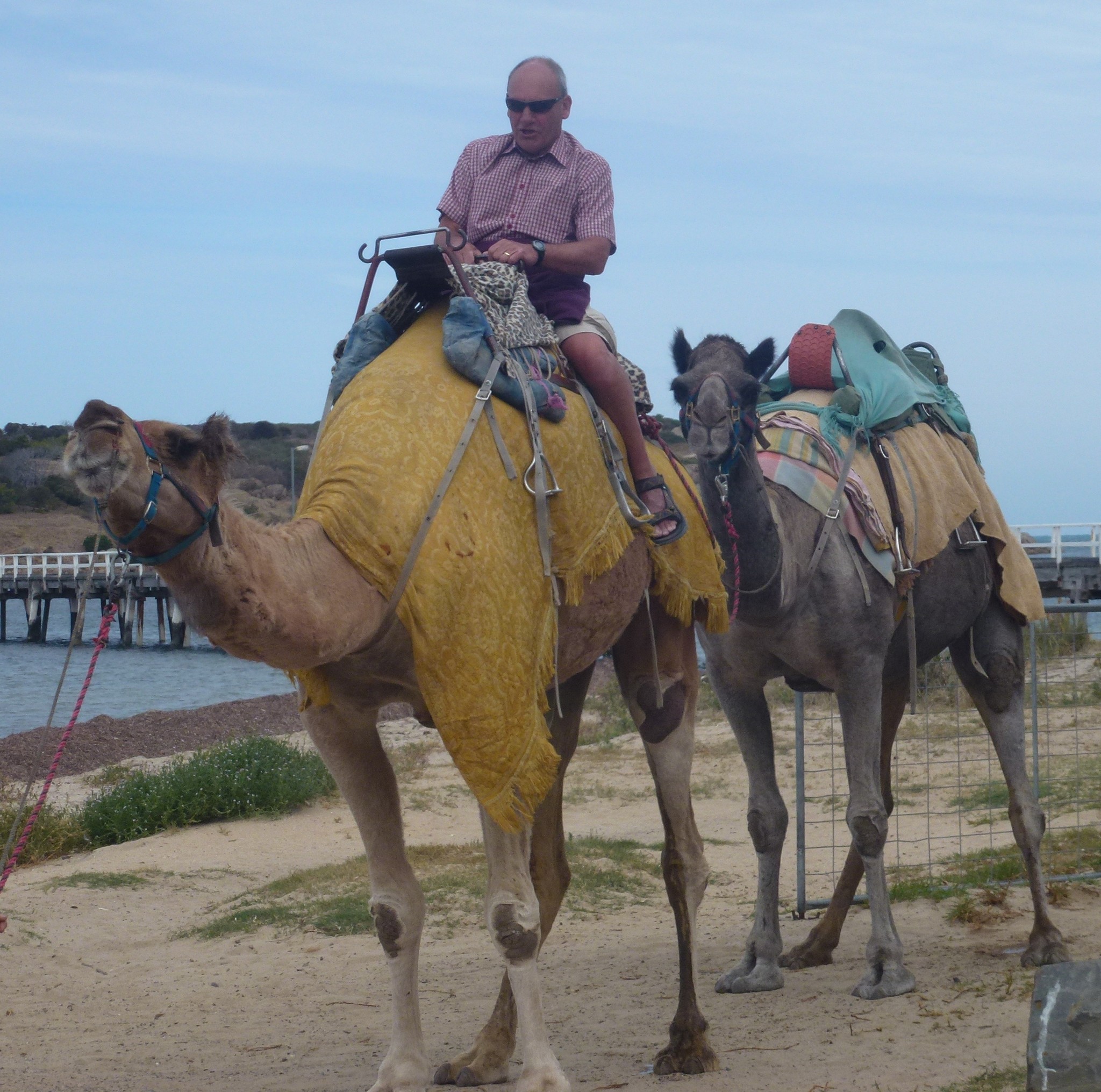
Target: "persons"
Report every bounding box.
[433,54,687,544]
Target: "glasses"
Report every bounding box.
[506,93,567,114]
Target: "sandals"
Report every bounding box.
[635,473,687,547]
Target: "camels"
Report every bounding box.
[63,297,719,1092]
[671,329,1072,998]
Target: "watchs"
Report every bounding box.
[527,240,546,268]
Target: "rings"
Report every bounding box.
[504,252,511,256]
